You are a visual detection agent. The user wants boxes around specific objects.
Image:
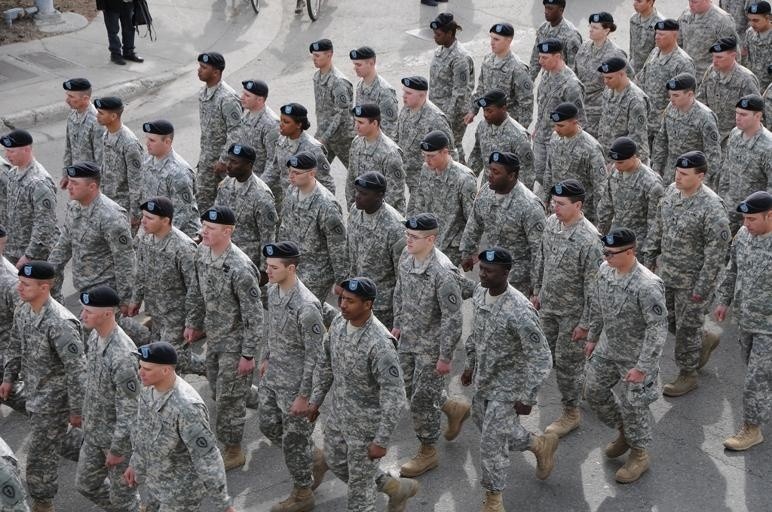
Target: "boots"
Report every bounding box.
[544,405,581,438]
[377,471,420,512]
[220,444,245,471]
[29,498,55,512]
[399,443,438,478]
[480,492,505,512]
[615,448,650,484]
[311,447,328,490]
[699,331,719,368]
[663,368,699,397]
[722,421,764,451]
[606,425,630,458]
[270,482,316,512]
[529,432,559,479]
[440,398,471,441]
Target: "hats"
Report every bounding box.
[198,52,225,68]
[80,287,119,307]
[67,161,100,177]
[401,76,428,90]
[139,196,174,217]
[200,205,237,226]
[589,12,614,23]
[748,1,770,14]
[420,129,448,151]
[478,246,512,265]
[536,38,563,53]
[0,130,33,147]
[489,151,520,169]
[228,143,256,162]
[351,104,380,118]
[262,240,301,258]
[736,191,772,214]
[430,13,453,30]
[666,72,695,90]
[674,150,706,168]
[285,151,318,169]
[309,39,332,53]
[93,97,122,111]
[602,227,635,247]
[142,119,174,135]
[280,103,307,119]
[478,88,505,107]
[341,277,377,299]
[354,171,387,192]
[489,22,514,37]
[709,37,735,53]
[550,179,586,198]
[62,78,91,91]
[242,79,268,95]
[607,136,637,160]
[405,213,439,230]
[736,94,763,111]
[597,57,626,74]
[654,19,679,30]
[138,341,178,365]
[17,260,54,279]
[350,46,374,60]
[550,102,579,122]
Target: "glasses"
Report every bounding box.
[405,233,431,240]
[602,247,633,256]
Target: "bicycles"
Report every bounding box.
[250,0,260,15]
[306,0,323,22]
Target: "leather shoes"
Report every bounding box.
[110,52,126,66]
[123,49,144,62]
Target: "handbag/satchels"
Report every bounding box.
[133,0,152,27]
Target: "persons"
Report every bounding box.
[0,0,772,510]
